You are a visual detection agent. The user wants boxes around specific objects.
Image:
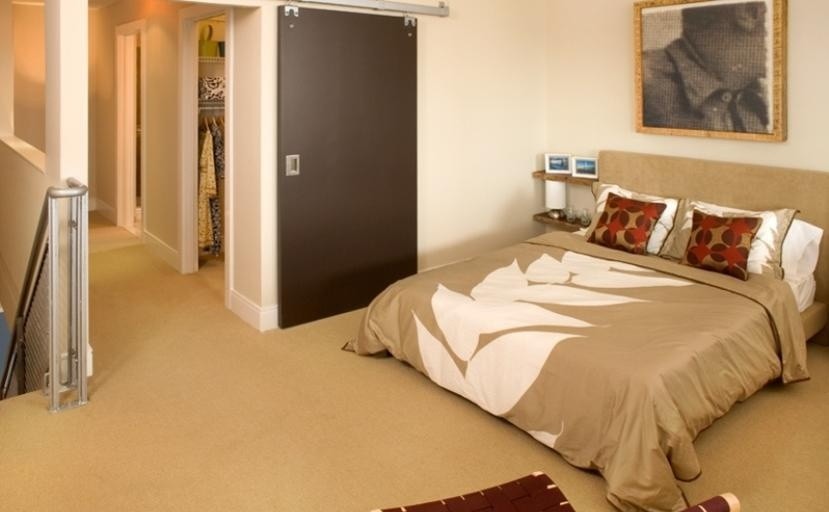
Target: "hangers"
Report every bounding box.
[200,103,224,131]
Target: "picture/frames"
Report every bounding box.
[632,0,788,142]
[545,153,572,173]
[572,157,599,179]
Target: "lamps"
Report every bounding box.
[545,182,566,219]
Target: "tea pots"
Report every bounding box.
[564,208,578,224]
[580,208,591,226]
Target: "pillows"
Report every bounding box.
[578,181,680,255]
[588,192,667,257]
[658,197,801,280]
[681,209,764,282]
[784,221,824,280]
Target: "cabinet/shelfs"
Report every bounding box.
[533,170,599,233]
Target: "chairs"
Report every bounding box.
[369,468,742,512]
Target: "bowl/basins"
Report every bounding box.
[198,41,217,57]
[218,40,224,56]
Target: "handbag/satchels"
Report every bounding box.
[197,24,219,56]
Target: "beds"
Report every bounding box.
[337,151,827,512]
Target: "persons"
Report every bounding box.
[641,4,770,132]
